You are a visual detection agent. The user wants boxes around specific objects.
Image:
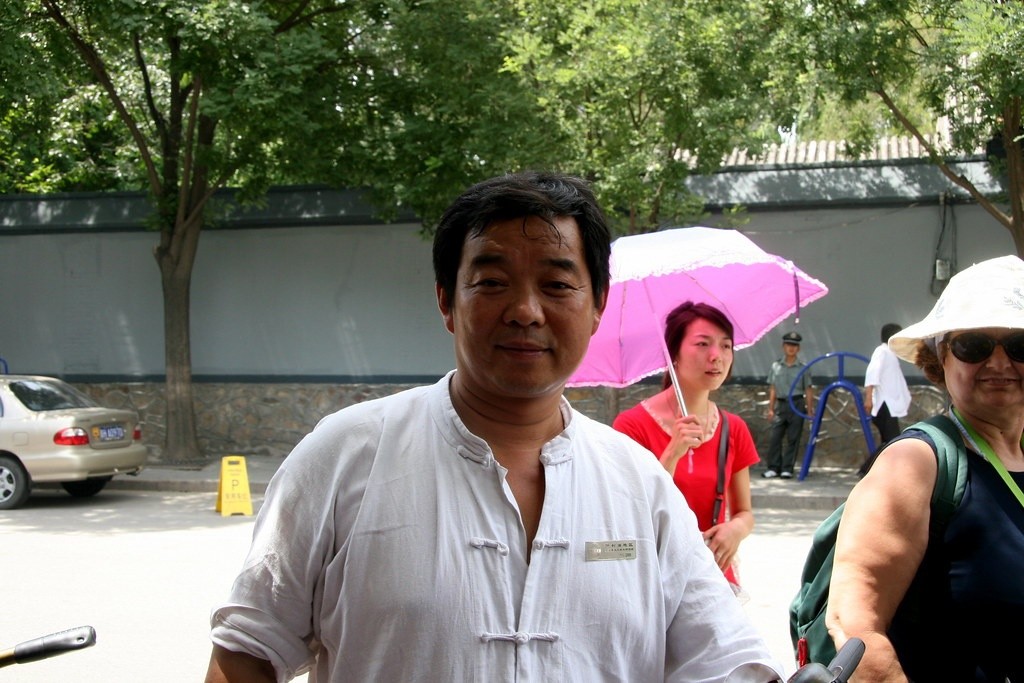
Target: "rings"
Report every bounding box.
[718,553,722,558]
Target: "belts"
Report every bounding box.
[776,395,803,403]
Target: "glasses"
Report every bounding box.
[945,332,1024,364]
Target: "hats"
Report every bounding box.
[782,332,802,345]
[887,255,1024,363]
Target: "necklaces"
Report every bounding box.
[665,388,709,432]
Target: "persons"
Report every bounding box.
[763,331,814,479]
[863,323,913,443]
[203,171,782,682]
[612,300,762,588]
[824,254,1024,683]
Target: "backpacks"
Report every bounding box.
[790,414,967,671]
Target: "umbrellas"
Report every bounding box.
[563,226,829,421]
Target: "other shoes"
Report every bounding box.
[761,470,781,478]
[780,471,793,478]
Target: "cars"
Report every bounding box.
[1,371,150,510]
